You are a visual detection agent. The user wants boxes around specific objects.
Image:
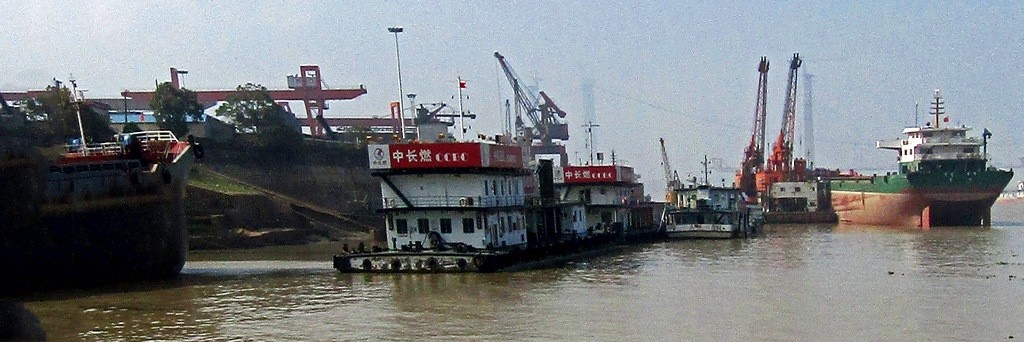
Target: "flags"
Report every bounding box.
[597,153,604,160]
[944,116,949,122]
[461,83,466,88]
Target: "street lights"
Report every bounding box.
[387,26,407,140]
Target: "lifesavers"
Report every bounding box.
[459,197,468,209]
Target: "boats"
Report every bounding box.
[659,137,743,239]
[333,50,671,275]
[0,72,205,295]
[813,88,1013,231]
[730,51,840,223]
